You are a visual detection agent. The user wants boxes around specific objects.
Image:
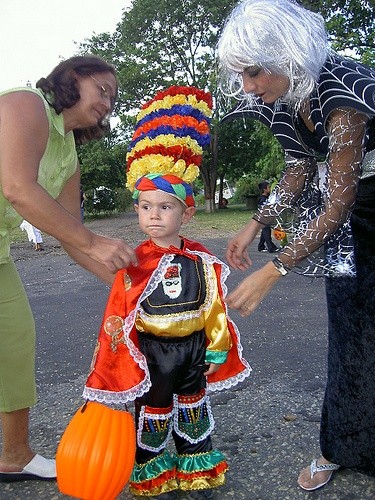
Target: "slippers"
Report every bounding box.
[298,458,340,491]
[0,453,57,483]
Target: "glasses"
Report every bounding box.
[87,73,115,107]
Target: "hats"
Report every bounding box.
[126,86,214,208]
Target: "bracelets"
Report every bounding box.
[273,259,288,276]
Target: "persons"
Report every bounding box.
[217,0,375,491]
[257,181,280,252]
[0,55,138,484]
[19,220,45,251]
[82,172,252,500]
